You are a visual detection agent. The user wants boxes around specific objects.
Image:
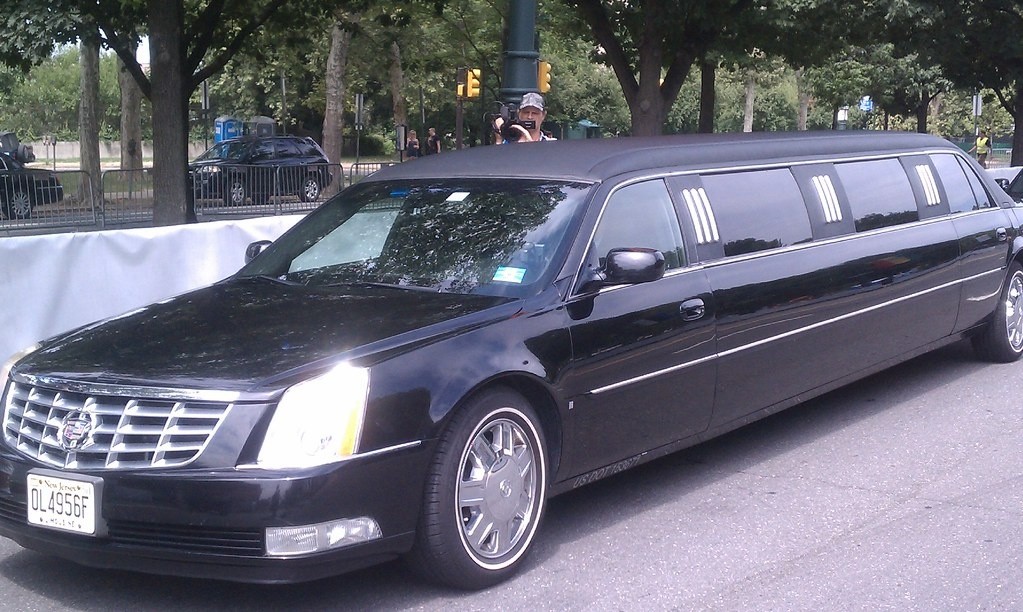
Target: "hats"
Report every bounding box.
[519,92,545,111]
[981,129,986,133]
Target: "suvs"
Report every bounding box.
[188,133,334,208]
[0,131,36,167]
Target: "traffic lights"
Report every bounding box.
[538,61,553,92]
[466,69,482,99]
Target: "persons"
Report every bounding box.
[496,92,549,146]
[968,130,991,168]
[544,131,552,138]
[427,128,441,154]
[406,130,420,159]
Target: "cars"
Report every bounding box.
[0,151,65,221]
[0,128,1023,592]
[993,166,1023,200]
